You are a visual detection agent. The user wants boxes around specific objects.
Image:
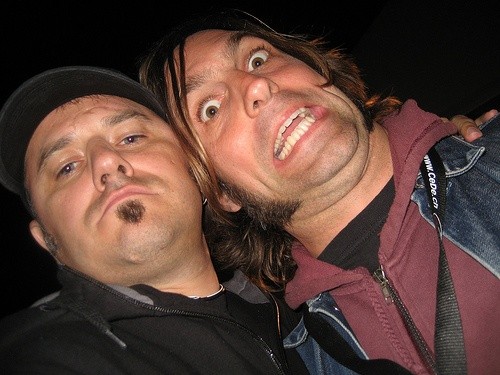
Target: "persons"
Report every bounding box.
[139,1,499,375]
[0,66,500,375]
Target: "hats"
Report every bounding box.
[2,63,169,201]
